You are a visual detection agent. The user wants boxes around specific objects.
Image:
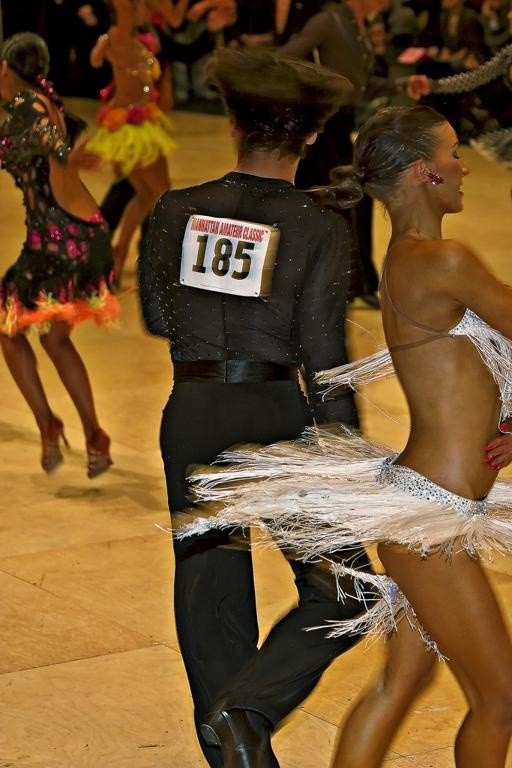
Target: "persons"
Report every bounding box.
[92,0,172,295]
[364,0,512,153]
[279,1,419,306]
[133,49,376,768]
[0,31,116,476]
[172,106,512,768]
[0,0,326,121]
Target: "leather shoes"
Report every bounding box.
[198,706,279,766]
[349,289,380,310]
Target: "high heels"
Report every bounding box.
[41,418,70,474]
[86,428,112,478]
